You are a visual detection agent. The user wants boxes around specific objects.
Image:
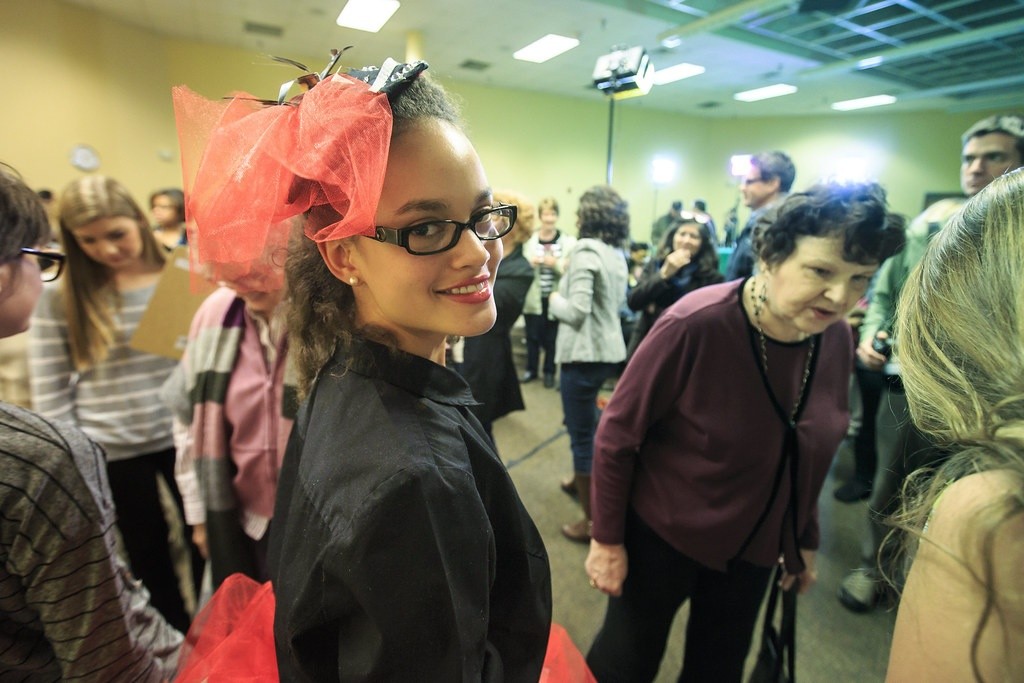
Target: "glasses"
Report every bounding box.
[742,176,766,186]
[360,201,519,256]
[16,245,67,283]
[679,209,717,240]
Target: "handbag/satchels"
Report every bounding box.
[750,562,801,683]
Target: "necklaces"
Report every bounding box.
[750,281,814,429]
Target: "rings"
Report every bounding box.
[590,579,597,589]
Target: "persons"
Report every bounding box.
[890,164,1024,683]
[267,61,554,683]
[443,149,798,544]
[583,179,903,683]
[832,115,1024,613]
[0,173,300,683]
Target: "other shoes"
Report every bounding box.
[833,473,871,503]
[836,562,888,611]
[562,471,589,495]
[543,372,556,389]
[519,370,539,384]
[561,517,590,544]
